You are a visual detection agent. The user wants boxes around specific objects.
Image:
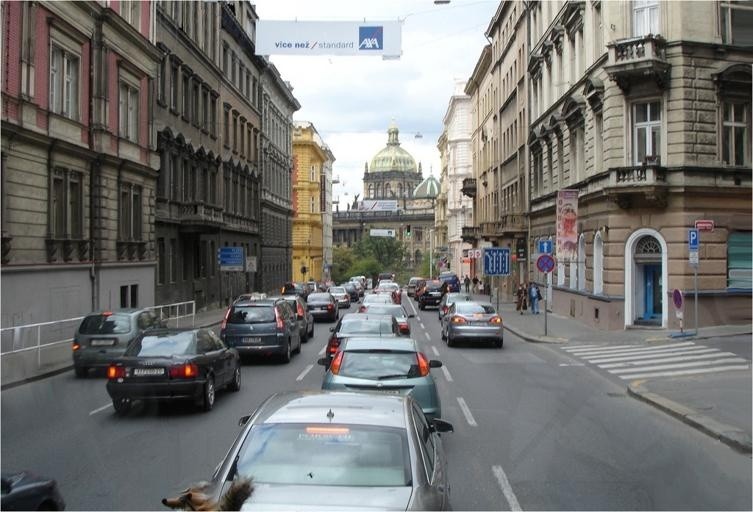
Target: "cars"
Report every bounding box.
[261,270,414,338]
[317,337,442,421]
[0,467,65,512]
[319,314,401,367]
[106,325,240,416]
[441,301,503,348]
[204,386,457,512]
[437,293,472,319]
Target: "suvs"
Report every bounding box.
[212,298,300,361]
[415,280,447,308]
[436,271,460,294]
[71,308,167,382]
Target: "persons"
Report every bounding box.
[528,283,540,315]
[516,284,527,315]
[464,275,470,293]
[472,275,479,294]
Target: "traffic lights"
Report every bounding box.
[407,224,410,235]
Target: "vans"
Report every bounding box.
[406,276,423,295]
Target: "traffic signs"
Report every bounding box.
[219,247,244,271]
